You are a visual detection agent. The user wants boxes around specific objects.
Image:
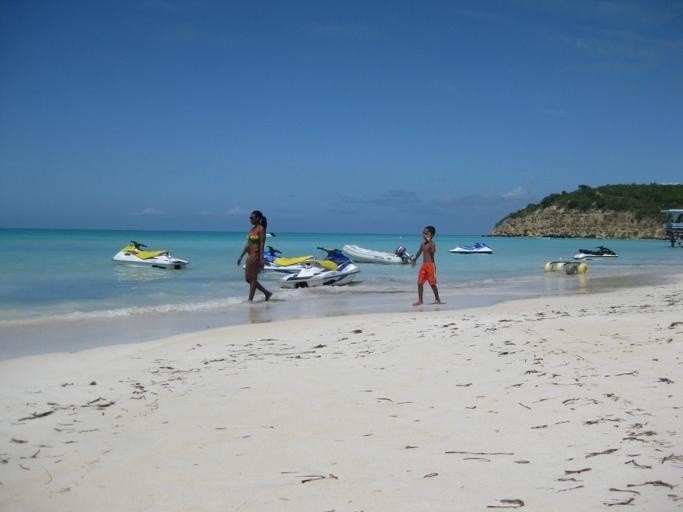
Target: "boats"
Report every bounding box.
[575,244,619,260]
[112,239,188,270]
[449,242,494,254]
[280,247,360,289]
[241,233,316,274]
[341,244,416,266]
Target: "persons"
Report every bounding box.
[236,212,272,304]
[409,227,443,306]
